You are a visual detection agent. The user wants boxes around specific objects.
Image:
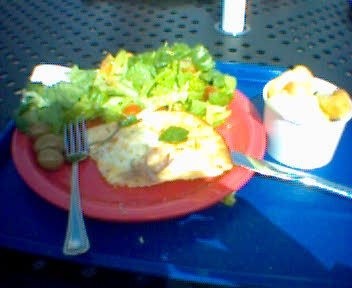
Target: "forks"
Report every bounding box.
[63,118,91,256]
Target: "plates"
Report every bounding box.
[10,74,267,224]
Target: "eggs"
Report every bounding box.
[81,109,233,189]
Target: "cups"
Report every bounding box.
[262,77,352,170]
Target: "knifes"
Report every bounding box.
[231,151,352,200]
